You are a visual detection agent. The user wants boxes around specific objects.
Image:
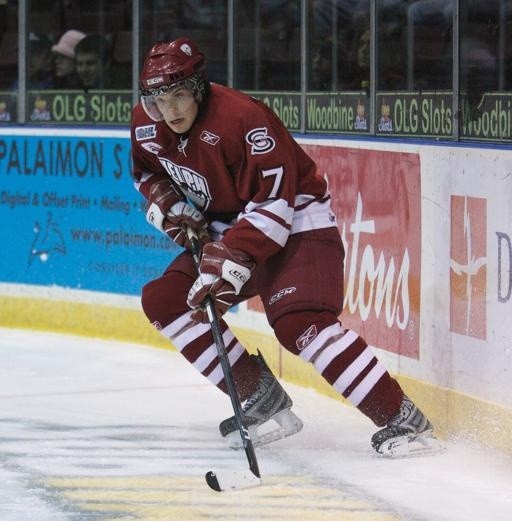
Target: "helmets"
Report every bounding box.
[137,36,207,123]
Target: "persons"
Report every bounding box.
[128,36,436,452]
[1,0,512,91]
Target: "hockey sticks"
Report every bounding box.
[186,226,261,491]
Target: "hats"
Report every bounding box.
[12,28,86,59]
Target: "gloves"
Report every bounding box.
[187,243,256,324]
[143,180,213,252]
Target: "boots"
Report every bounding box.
[372,379,435,453]
[219,348,293,437]
